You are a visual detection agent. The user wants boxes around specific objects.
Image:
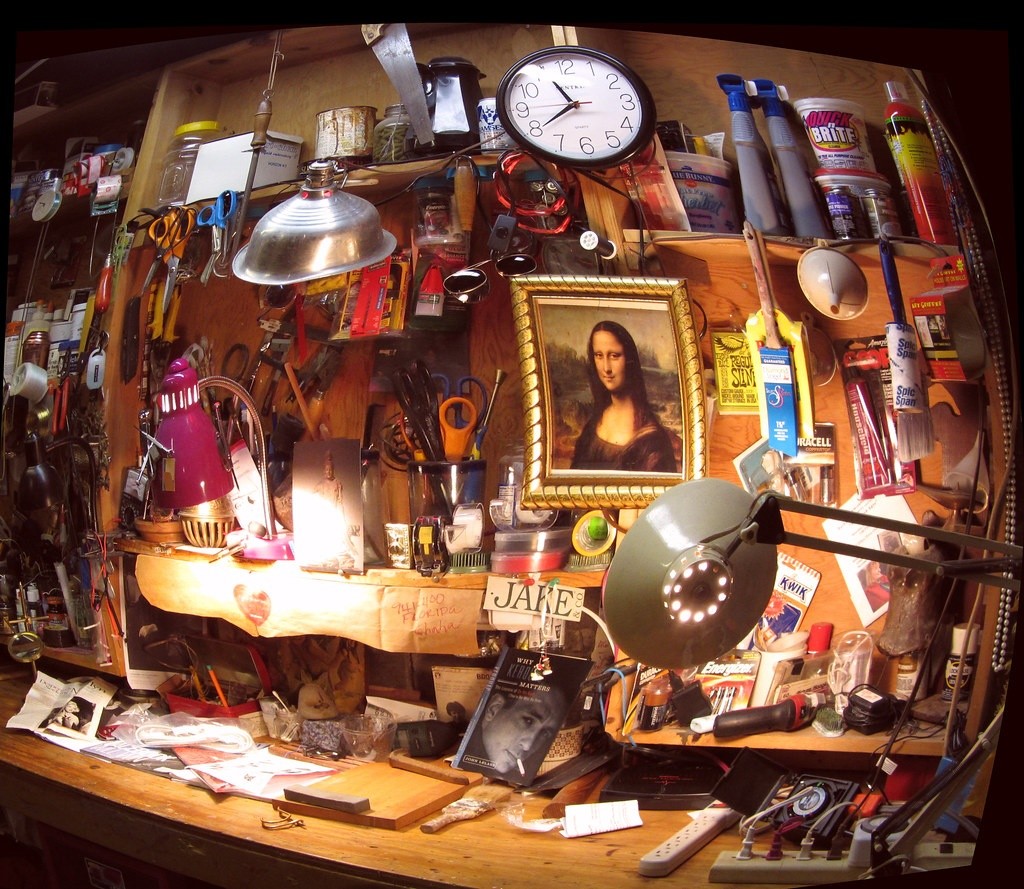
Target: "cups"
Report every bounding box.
[340,713,397,762]
[408,459,484,568]
[274,709,302,742]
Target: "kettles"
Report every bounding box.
[404,57,486,159]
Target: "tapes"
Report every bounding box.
[93,174,122,204]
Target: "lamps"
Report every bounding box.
[603,478,1024,671]
[232,161,397,287]
[132,358,292,558]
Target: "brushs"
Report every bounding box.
[420,783,515,834]
[882,240,935,463]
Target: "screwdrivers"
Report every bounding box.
[228,88,276,267]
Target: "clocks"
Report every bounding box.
[496,46,656,167]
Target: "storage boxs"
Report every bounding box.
[167,634,273,719]
[183,129,303,206]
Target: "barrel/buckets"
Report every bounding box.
[663,151,738,234]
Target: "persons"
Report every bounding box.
[445,702,472,731]
[51,700,83,728]
[481,662,566,772]
[314,451,347,525]
[866,563,892,611]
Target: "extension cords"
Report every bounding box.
[910,842,976,871]
[638,800,742,877]
[708,849,873,885]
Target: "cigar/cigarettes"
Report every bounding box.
[517,759,525,776]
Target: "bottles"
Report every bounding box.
[361,448,388,567]
[500,443,559,533]
[162,122,219,201]
[375,103,419,165]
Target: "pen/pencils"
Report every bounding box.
[206,664,228,708]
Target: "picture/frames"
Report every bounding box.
[509,273,709,510]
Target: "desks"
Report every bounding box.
[0,670,807,889]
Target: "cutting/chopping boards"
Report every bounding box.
[273,756,482,829]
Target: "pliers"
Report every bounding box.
[48,343,73,437]
[149,275,183,383]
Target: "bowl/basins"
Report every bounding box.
[135,515,185,541]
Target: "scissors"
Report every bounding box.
[141,204,198,313]
[389,357,489,524]
[196,189,237,264]
